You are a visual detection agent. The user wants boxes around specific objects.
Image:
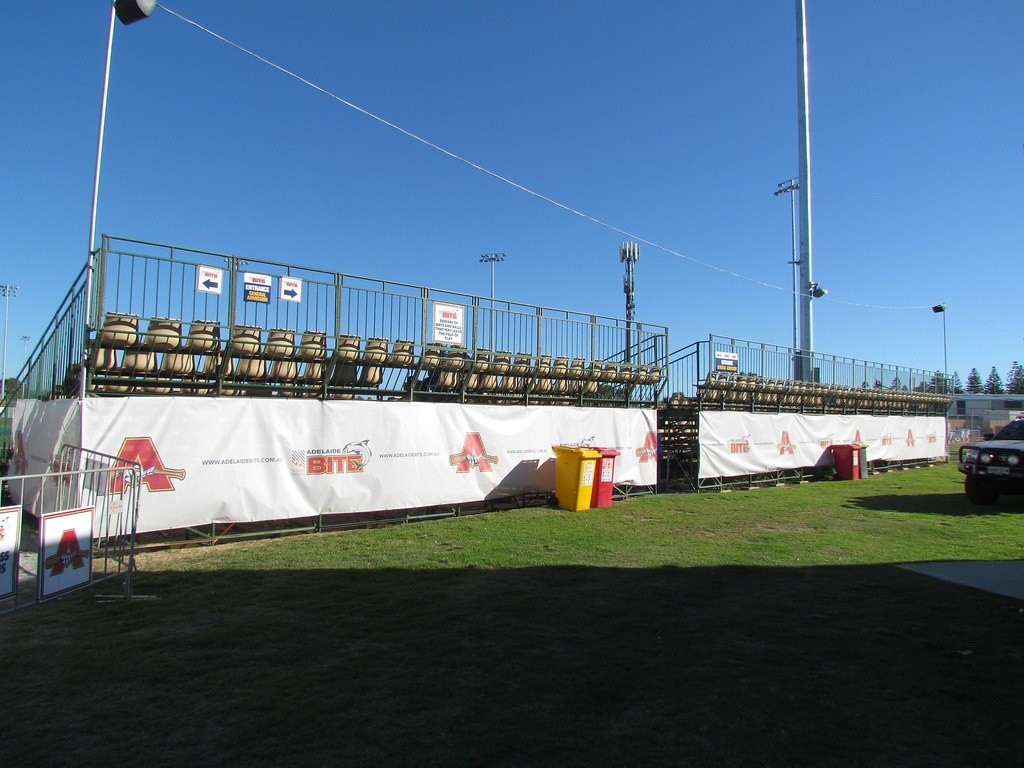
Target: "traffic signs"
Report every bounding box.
[279,276,302,302]
[196,265,223,294]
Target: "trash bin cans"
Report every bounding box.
[830,444,861,480]
[580,446,622,508]
[852,443,869,478]
[547,446,602,511]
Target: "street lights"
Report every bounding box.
[932,302,947,396]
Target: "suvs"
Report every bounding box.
[958,412,1024,501]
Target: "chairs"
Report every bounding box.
[55,310,665,404]
[672,372,955,418]
